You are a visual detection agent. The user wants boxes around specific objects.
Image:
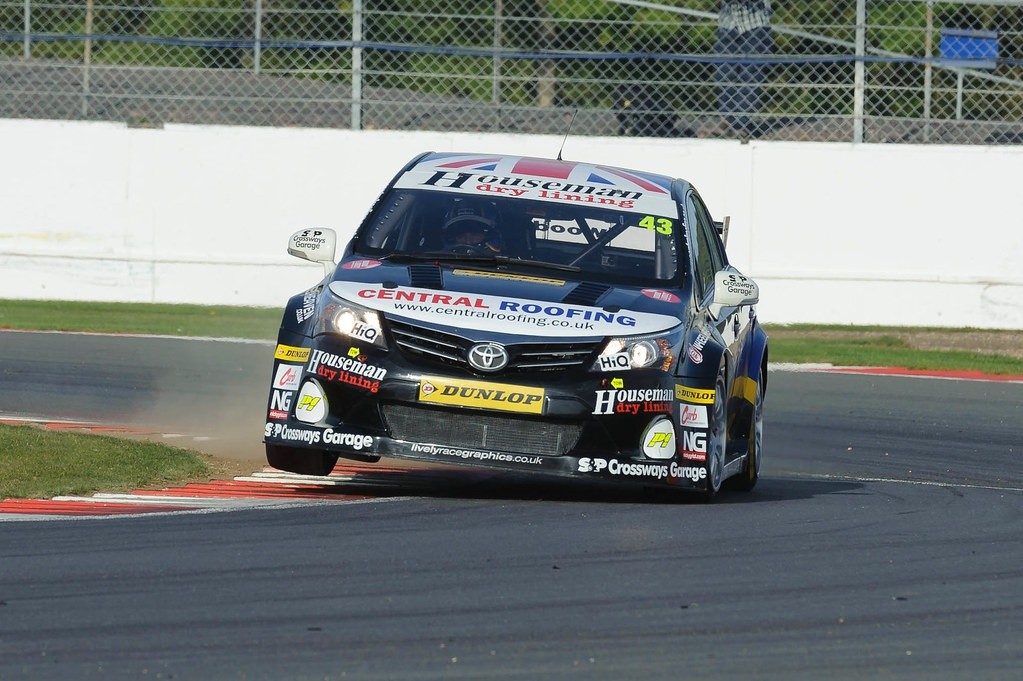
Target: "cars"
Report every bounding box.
[262,151,771,499]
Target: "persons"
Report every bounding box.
[441,198,509,261]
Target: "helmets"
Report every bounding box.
[441,196,503,255]
